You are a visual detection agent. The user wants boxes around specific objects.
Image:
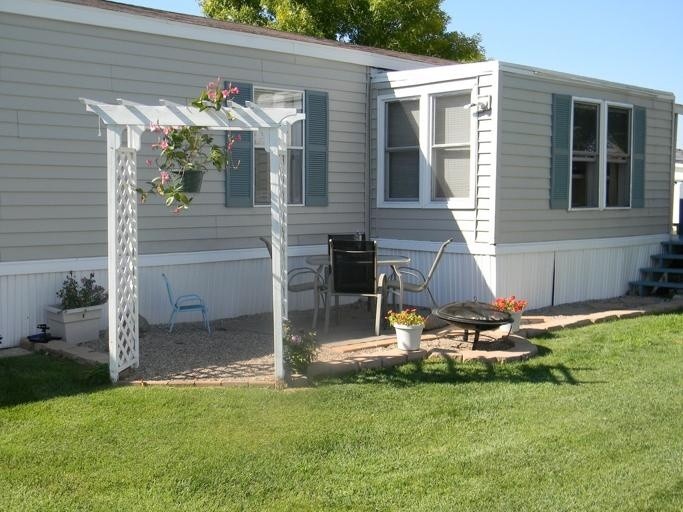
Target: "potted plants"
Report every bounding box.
[43,269,109,346]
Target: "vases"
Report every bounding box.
[499,311,522,333]
[170,169,204,194]
[392,324,424,350]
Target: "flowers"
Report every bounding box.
[384,307,426,327]
[129,75,241,215]
[495,296,528,313]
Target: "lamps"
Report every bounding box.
[464,101,488,112]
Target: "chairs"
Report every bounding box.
[382,237,455,332]
[161,273,212,335]
[325,239,388,337]
[327,233,371,312]
[257,236,328,329]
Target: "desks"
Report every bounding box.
[306,254,410,325]
